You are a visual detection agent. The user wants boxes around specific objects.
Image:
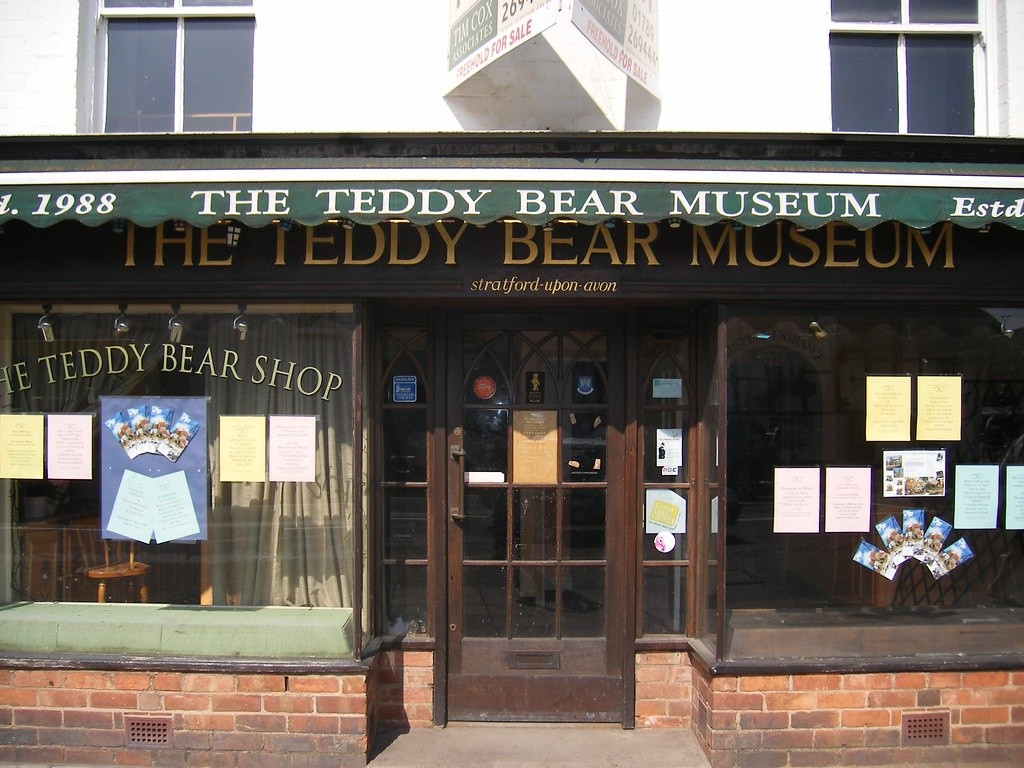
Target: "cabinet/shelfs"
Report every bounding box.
[15,497,101,602]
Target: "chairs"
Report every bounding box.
[87,536,151,603]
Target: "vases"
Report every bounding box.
[23,495,48,518]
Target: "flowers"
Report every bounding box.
[17,462,72,498]
